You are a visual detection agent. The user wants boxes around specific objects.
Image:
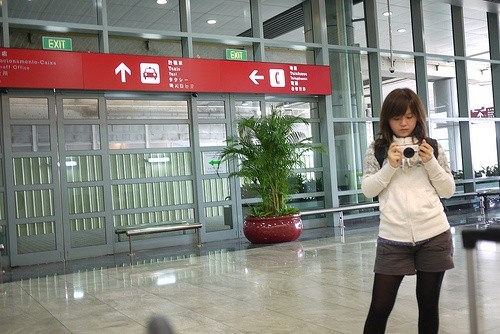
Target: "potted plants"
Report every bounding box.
[219,106,327,244]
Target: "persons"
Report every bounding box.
[361,88,455,334]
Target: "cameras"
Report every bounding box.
[395,145,424,161]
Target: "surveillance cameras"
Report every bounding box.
[389,67,396,73]
